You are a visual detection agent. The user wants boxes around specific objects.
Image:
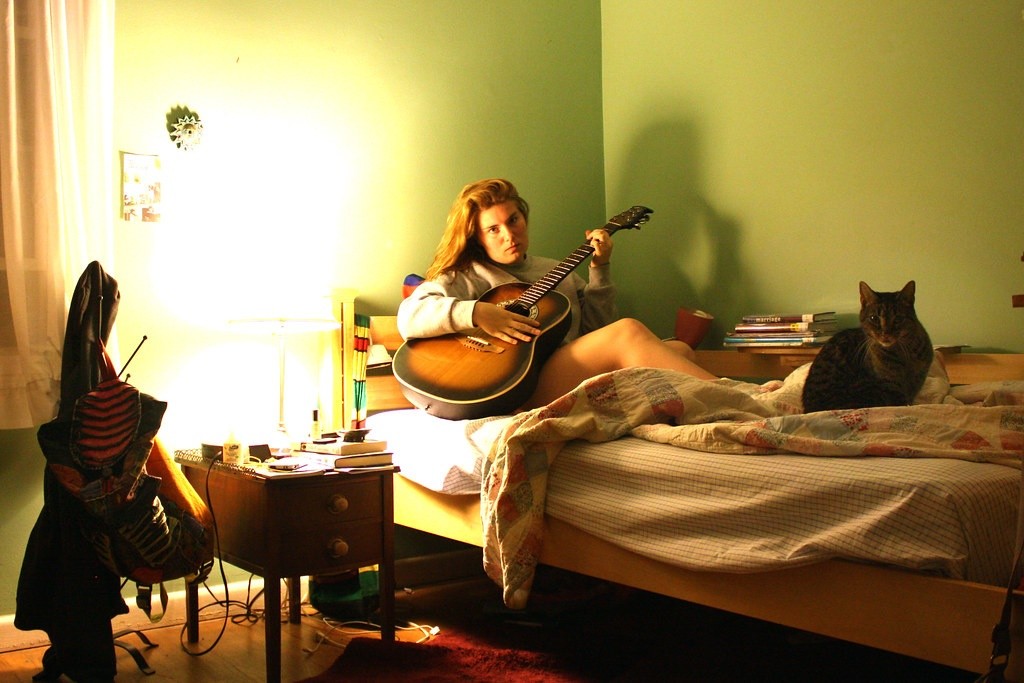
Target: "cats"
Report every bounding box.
[800,280,934,415]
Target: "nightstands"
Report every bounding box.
[174,445,401,683]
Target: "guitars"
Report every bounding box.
[390,203,655,422]
[95,335,216,587]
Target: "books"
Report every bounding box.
[723,311,841,350]
[293,439,395,468]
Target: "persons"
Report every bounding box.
[398,179,721,412]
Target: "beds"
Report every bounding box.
[329,296,1023,683]
